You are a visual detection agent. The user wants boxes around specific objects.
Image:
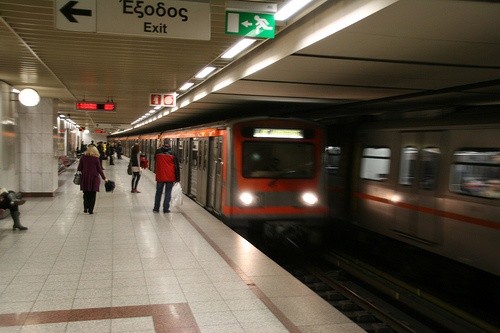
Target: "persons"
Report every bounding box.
[77,145,108,215]
[0,186,27,231]
[77,140,122,170]
[130,143,149,193]
[153,138,180,213]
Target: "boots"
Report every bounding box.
[7,193,25,206]
[11,211,28,231]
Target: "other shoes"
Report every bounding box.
[131,188,141,193]
[153,208,159,212]
[163,210,171,213]
[84,209,87,213]
[88,209,93,214]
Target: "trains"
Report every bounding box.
[106,115,334,240]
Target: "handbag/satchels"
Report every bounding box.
[170,182,183,207]
[128,160,133,175]
[73,171,81,185]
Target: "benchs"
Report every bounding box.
[58,159,78,174]
